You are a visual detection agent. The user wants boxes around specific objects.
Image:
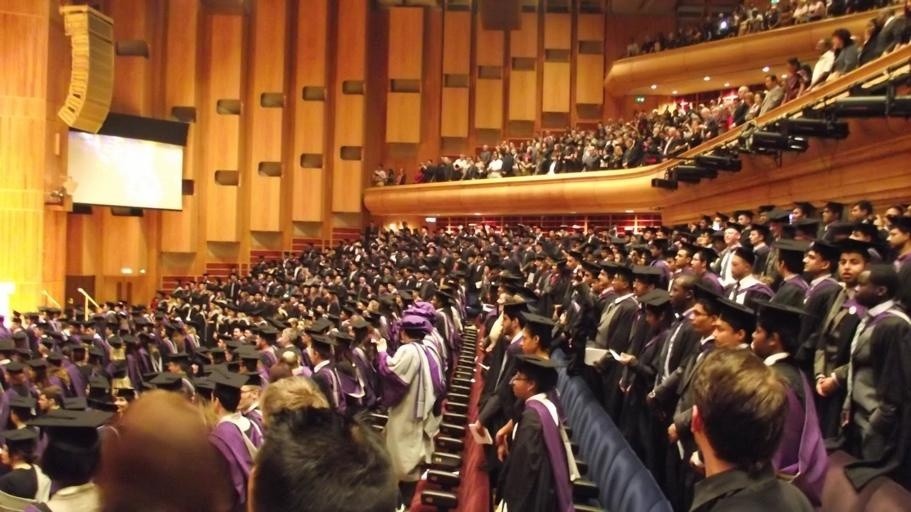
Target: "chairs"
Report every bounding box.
[551,346,674,509]
[403,312,488,511]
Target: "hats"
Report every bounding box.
[712,199,876,253]
[581,259,671,306]
[0,301,148,450]
[395,284,454,333]
[142,328,279,393]
[515,353,561,379]
[693,282,810,334]
[308,319,370,348]
[609,237,717,259]
[505,302,554,330]
